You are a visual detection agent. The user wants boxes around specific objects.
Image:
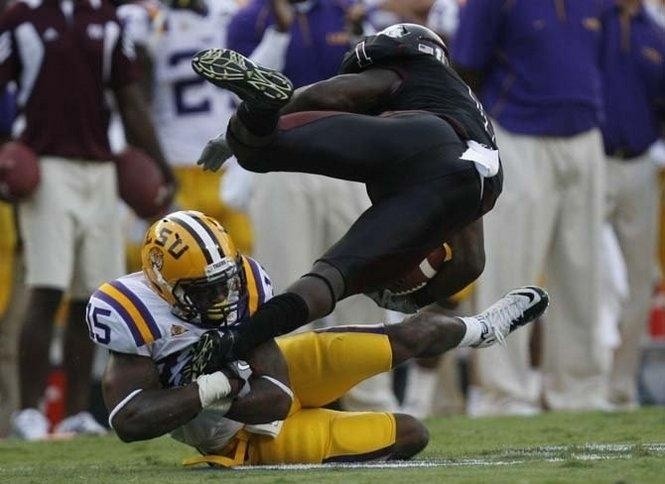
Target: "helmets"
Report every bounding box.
[143,210,250,328]
[379,24,451,65]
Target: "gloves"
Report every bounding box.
[197,136,231,173]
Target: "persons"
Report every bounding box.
[2,1,665,442]
[85,211,549,468]
[179,24,504,384]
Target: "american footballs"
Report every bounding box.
[382,242,453,296]
[115,148,167,217]
[1,142,40,197]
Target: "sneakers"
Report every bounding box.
[190,48,294,109]
[162,329,236,389]
[467,286,550,349]
